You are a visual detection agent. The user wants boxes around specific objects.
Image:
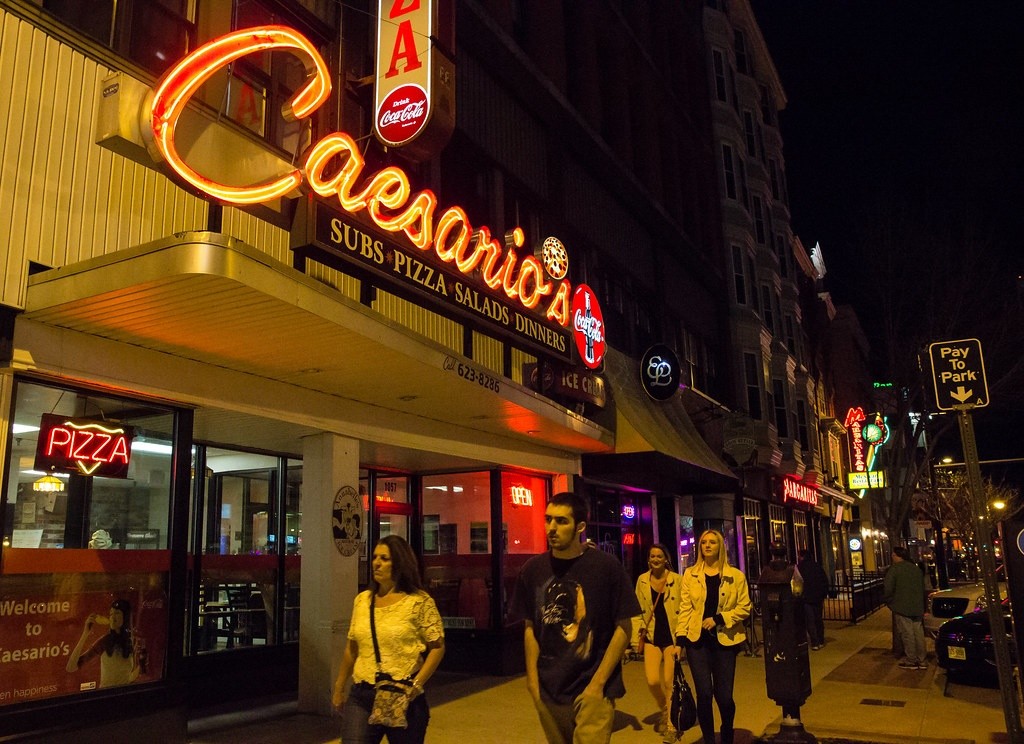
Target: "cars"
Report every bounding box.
[934,598,1018,670]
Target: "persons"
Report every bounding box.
[796,549,829,651]
[524,492,633,744]
[672,528,753,744]
[635,540,684,744]
[66,600,147,685]
[331,534,447,744]
[882,547,931,670]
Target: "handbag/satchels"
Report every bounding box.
[791,565,803,594]
[369,671,416,728]
[636,628,647,655]
[670,659,696,733]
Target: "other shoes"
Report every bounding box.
[820,644,824,648]
[920,662,928,669]
[664,730,682,743]
[898,660,918,669]
[812,646,818,649]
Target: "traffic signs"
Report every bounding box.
[929,339,989,411]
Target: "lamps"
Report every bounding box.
[33,472,64,493]
[192,447,213,479]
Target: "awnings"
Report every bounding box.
[595,343,740,495]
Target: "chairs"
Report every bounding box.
[199,579,253,648]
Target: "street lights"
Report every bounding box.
[931,457,952,589]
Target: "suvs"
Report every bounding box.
[923,563,1007,632]
